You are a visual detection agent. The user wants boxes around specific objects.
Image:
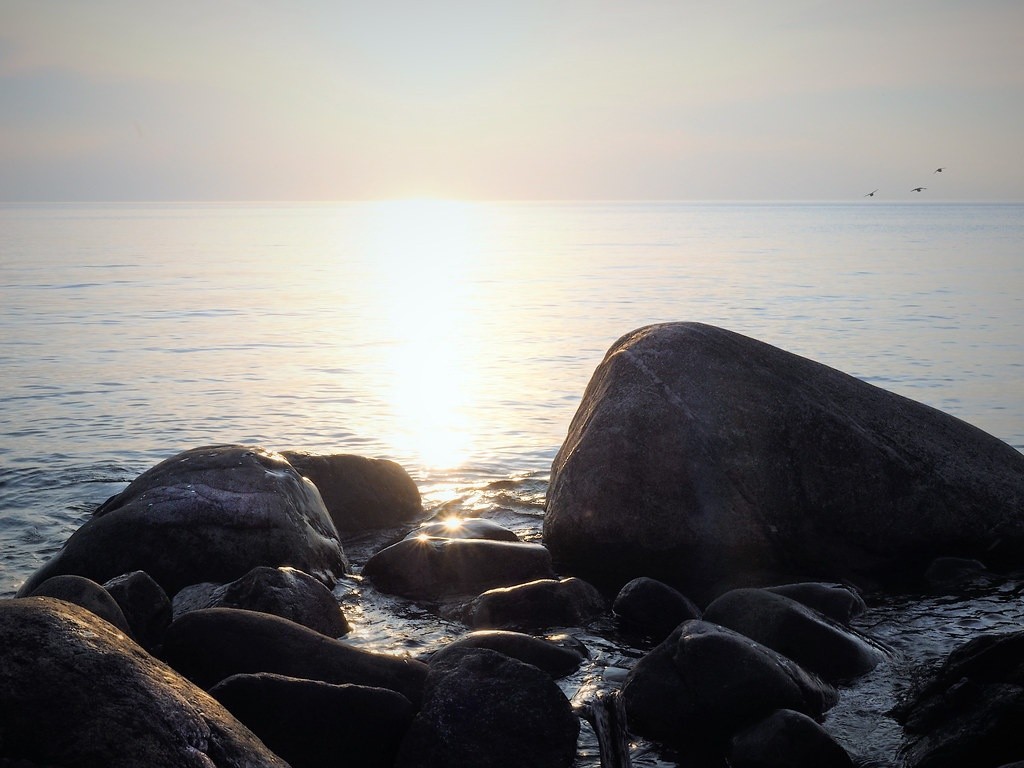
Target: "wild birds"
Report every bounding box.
[863,188,880,198]
[933,167,947,173]
[910,187,927,192]
